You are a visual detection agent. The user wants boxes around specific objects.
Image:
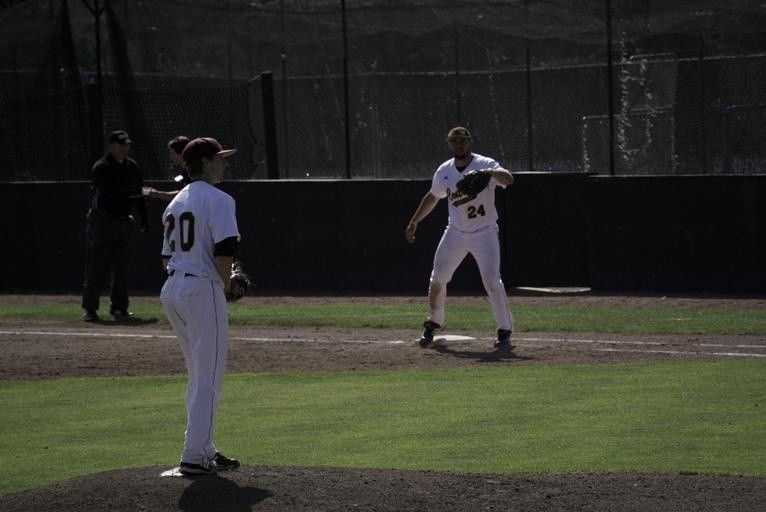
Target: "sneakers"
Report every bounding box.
[180,457,216,474]
[109,309,134,317]
[83,310,100,323]
[493,326,513,348]
[419,326,435,347]
[200,452,242,469]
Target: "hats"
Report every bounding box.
[107,131,132,144]
[182,138,236,161]
[447,126,473,141]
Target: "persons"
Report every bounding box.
[159,137,249,476]
[141,133,192,201]
[79,129,152,324]
[404,126,517,347]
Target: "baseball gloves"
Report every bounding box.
[454,167,492,198]
[225,270,255,298]
